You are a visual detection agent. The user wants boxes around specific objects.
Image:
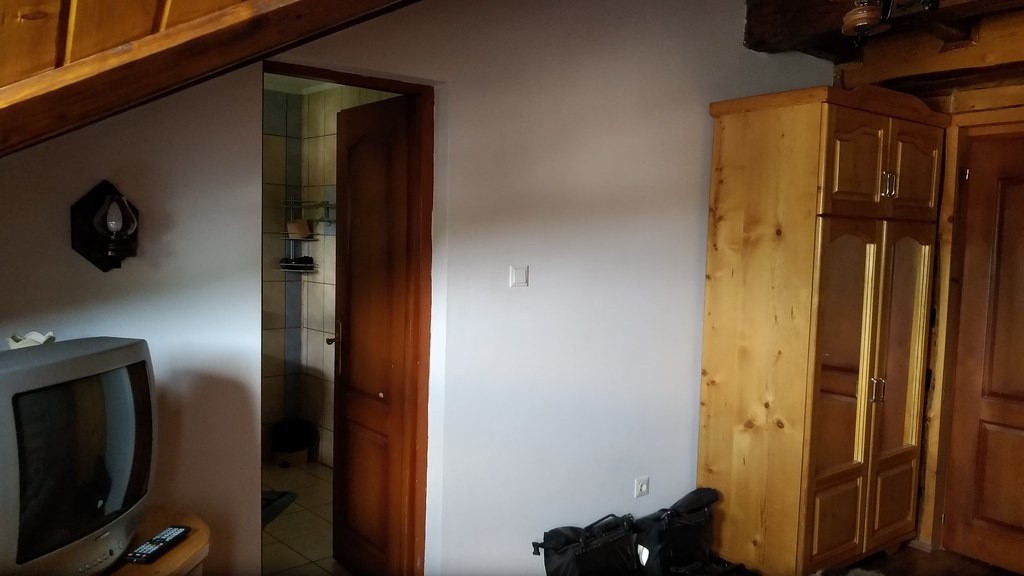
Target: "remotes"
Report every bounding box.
[126,525,191,564]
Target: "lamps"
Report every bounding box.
[72,179,141,272]
[842,0,941,47]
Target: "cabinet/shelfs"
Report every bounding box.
[279,200,319,273]
[0,0,421,159]
[697,83,953,576]
[106,508,211,576]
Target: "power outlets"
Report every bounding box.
[634,476,649,498]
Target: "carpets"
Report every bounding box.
[263,489,298,529]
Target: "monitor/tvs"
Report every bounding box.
[0,337,159,576]
